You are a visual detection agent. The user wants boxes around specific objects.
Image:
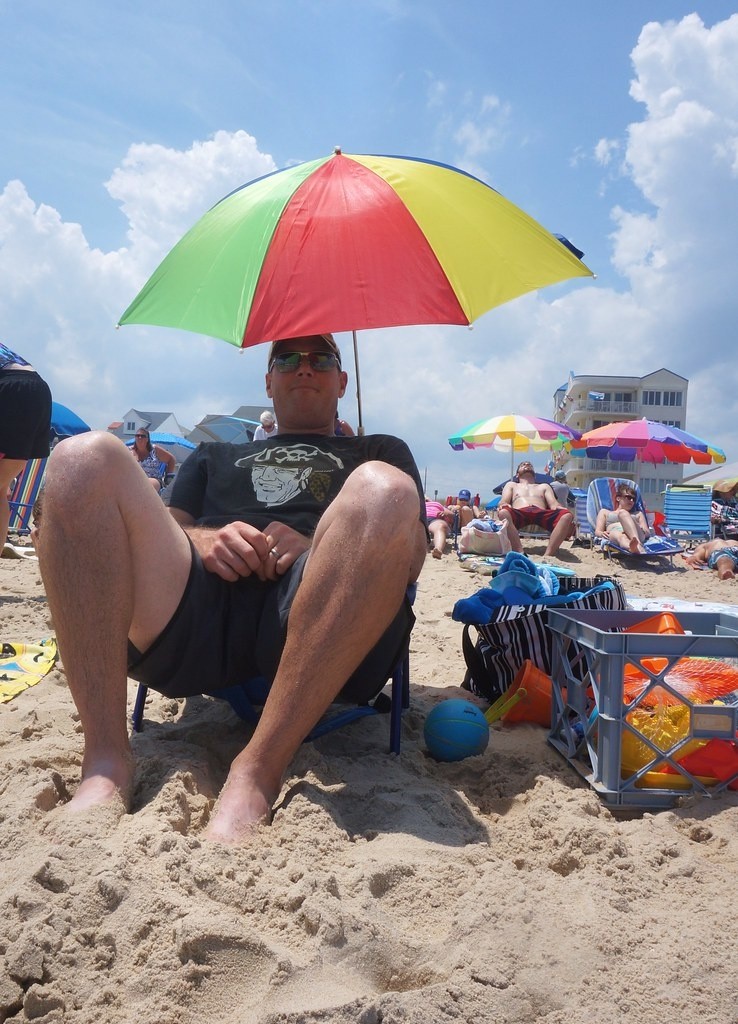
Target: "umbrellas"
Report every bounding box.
[195,415,261,445]
[49,402,91,440]
[581,416,727,485]
[449,413,582,484]
[115,146,596,440]
[124,433,196,463]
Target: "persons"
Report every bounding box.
[720,490,736,503]
[448,490,488,533]
[130,427,174,495]
[334,410,355,438]
[498,461,573,557]
[550,471,569,508]
[38,332,427,851]
[0,343,52,557]
[424,497,454,559]
[253,411,278,441]
[687,537,738,580]
[595,484,650,554]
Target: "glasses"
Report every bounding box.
[459,498,467,502]
[135,434,146,439]
[269,351,342,373]
[621,495,636,502]
[261,425,272,429]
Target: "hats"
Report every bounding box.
[459,490,471,500]
[267,333,341,371]
[555,471,566,479]
[711,478,738,498]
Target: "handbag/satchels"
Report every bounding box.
[462,574,633,720]
[458,519,512,556]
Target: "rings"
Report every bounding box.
[271,548,281,560]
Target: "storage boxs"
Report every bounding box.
[546,607,738,809]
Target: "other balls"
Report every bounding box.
[423,699,489,762]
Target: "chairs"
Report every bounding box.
[7,447,713,756]
